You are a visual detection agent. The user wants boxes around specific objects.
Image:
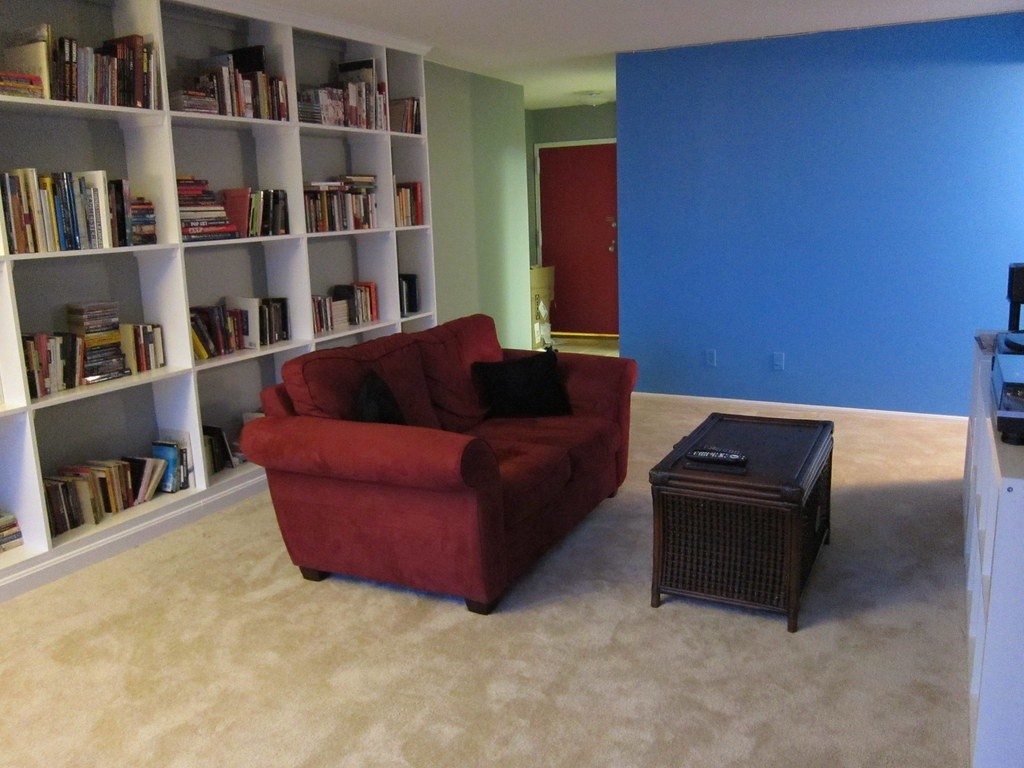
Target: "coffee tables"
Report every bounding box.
[647,413,835,633]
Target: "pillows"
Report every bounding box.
[350,366,410,427]
[472,343,574,418]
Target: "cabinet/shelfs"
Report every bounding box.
[963,329,1024,767]
[0,0,439,610]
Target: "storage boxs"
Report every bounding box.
[530,265,555,348]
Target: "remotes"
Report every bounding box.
[683,446,748,475]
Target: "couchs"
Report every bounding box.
[240,312,638,615]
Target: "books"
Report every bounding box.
[203,407,266,476]
[389,97,420,134]
[400,273,419,317]
[0,510,24,552]
[297,58,387,130]
[170,45,289,122]
[393,174,423,227]
[44,429,196,537]
[304,175,378,233]
[21,301,165,398]
[189,295,292,360]
[311,282,379,332]
[177,175,290,242]
[0,167,157,254]
[1,23,160,110]
